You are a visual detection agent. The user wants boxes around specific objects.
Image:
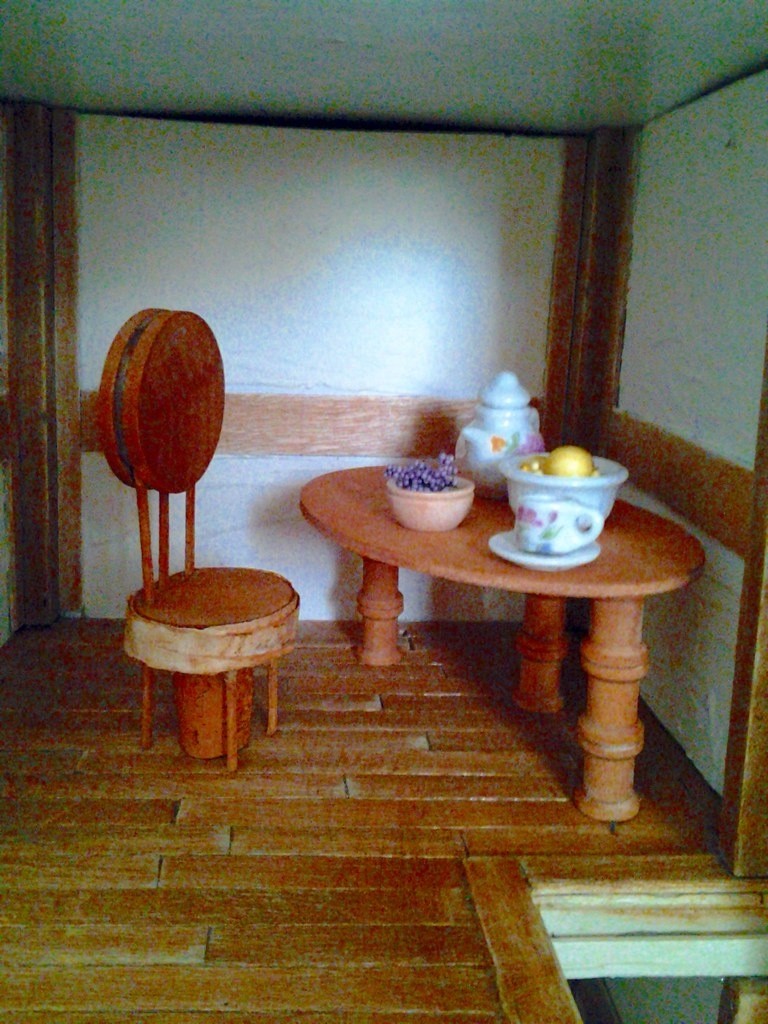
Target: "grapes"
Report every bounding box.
[383,448,457,492]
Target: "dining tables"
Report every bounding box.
[300,462,707,823]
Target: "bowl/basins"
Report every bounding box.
[381,478,476,532]
[502,451,628,525]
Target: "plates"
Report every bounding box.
[489,528,602,571]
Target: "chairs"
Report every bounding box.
[96,309,300,774]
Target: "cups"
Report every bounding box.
[516,493,603,555]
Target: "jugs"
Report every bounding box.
[455,371,546,502]
